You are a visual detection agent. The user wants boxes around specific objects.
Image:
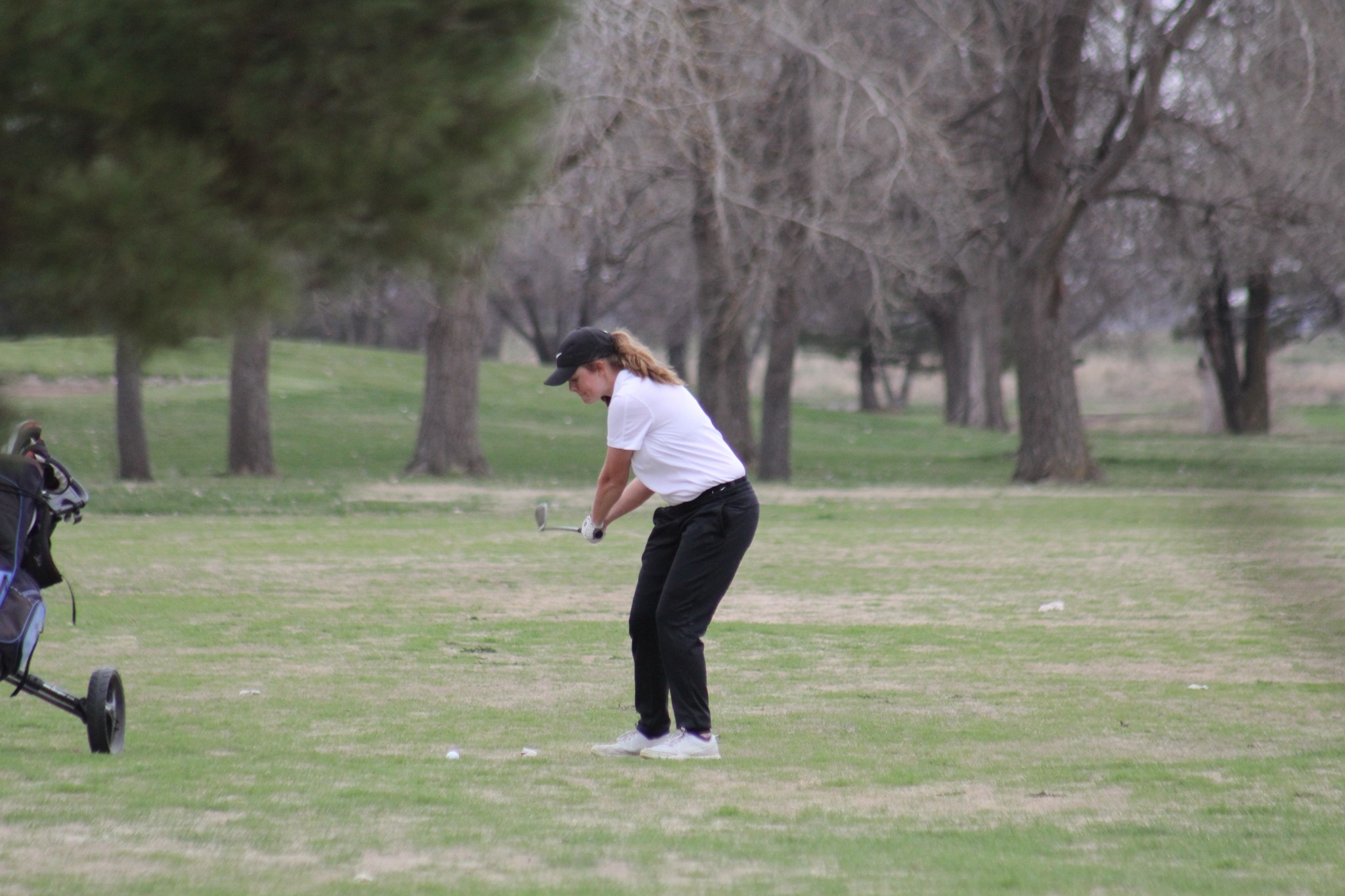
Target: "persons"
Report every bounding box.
[542,325,761,761]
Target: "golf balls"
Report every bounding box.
[446,752,460,760]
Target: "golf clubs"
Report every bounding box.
[534,502,603,539]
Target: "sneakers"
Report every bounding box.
[591,729,671,757]
[640,727,721,759]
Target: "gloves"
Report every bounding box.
[581,514,606,543]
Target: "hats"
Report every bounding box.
[544,326,615,386]
